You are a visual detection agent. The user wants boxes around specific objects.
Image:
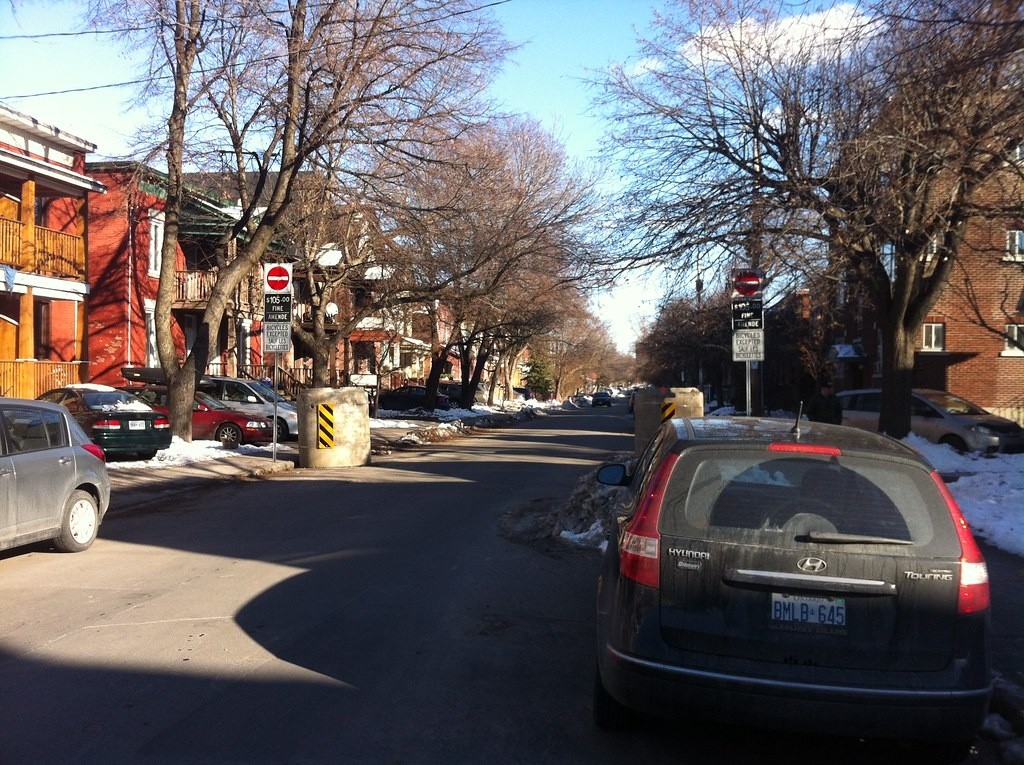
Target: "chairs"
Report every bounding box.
[20,421,50,452]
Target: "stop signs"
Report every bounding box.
[263,263,293,293]
[731,267,765,298]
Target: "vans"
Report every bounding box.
[438,382,486,406]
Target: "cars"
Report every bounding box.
[31,384,174,459]
[377,385,452,412]
[837,387,1023,458]
[592,392,613,407]
[0,398,111,555]
[626,389,637,411]
[513,387,537,400]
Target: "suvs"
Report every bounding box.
[596,415,998,744]
[188,374,301,443]
[111,367,274,450]
